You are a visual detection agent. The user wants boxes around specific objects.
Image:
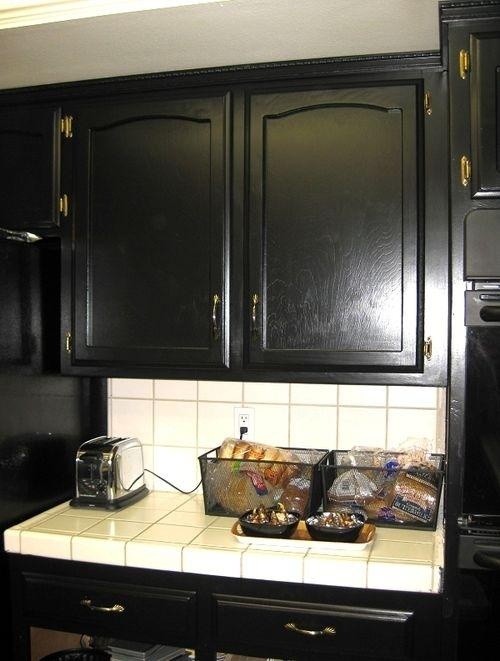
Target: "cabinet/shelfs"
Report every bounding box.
[66,77,426,376]
[437,23,500,203]
[8,568,431,661]
[1,104,63,241]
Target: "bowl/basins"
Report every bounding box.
[238,509,301,540]
[305,511,365,544]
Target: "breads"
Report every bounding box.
[326,464,439,525]
[212,440,310,513]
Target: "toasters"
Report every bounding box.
[69,436,149,511]
